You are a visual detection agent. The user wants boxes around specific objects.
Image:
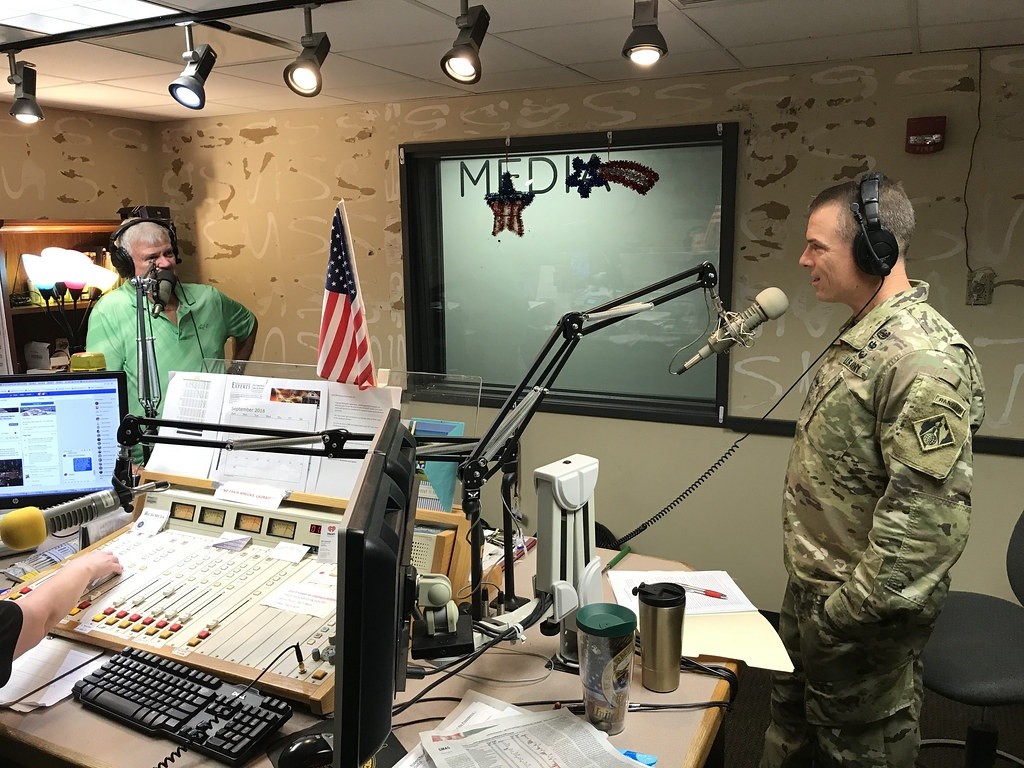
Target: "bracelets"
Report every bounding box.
[230,364,244,375]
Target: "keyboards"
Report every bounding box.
[72,647,293,768]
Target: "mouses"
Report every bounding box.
[278,732,333,768]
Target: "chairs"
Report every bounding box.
[918,508,1024,768]
[532,521,623,552]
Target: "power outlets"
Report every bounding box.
[963,270,994,306]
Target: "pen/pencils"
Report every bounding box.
[562,702,642,711]
[674,583,728,600]
[601,546,631,574]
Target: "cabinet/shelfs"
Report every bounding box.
[0,218,124,376]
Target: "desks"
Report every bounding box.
[0,545,738,768]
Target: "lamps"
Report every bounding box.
[440,0,492,86]
[22,246,119,356]
[5,48,45,125]
[168,21,218,110]
[282,3,333,98]
[621,0,668,67]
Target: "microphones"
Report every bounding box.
[677,287,788,376]
[153,270,177,319]
[0,481,170,550]
[850,202,891,276]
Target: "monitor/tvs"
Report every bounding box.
[331,408,417,768]
[0,370,129,510]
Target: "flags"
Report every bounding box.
[317,206,374,390]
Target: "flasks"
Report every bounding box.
[577,603,637,735]
[632,581,686,692]
[71,353,105,373]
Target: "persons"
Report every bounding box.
[86,217,258,476]
[0,551,122,689]
[757,176,985,768]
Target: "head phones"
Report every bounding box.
[854,171,899,277]
[109,217,179,277]
